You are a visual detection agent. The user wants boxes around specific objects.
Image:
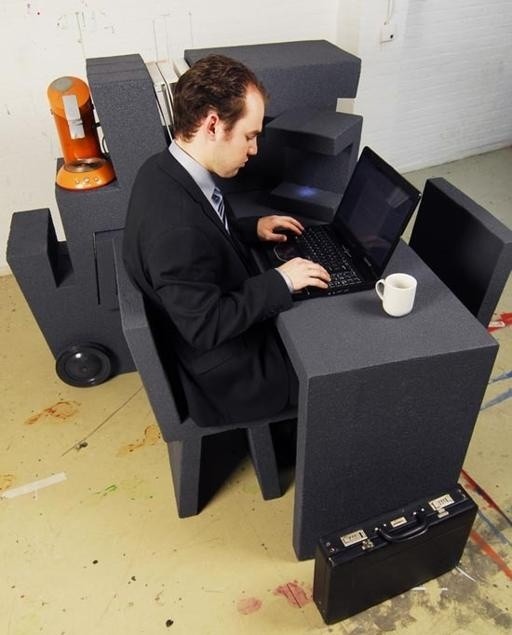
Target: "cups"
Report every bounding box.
[374,273,419,318]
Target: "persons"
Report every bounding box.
[121,53,332,429]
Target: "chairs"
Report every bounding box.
[409,178,512,329]
[113,236,298,518]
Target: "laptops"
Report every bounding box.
[265,145,421,301]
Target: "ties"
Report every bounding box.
[212,188,231,236]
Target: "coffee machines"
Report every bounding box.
[44,75,114,190]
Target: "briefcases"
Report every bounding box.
[312,483,478,626]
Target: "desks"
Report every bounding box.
[224,189,499,560]
